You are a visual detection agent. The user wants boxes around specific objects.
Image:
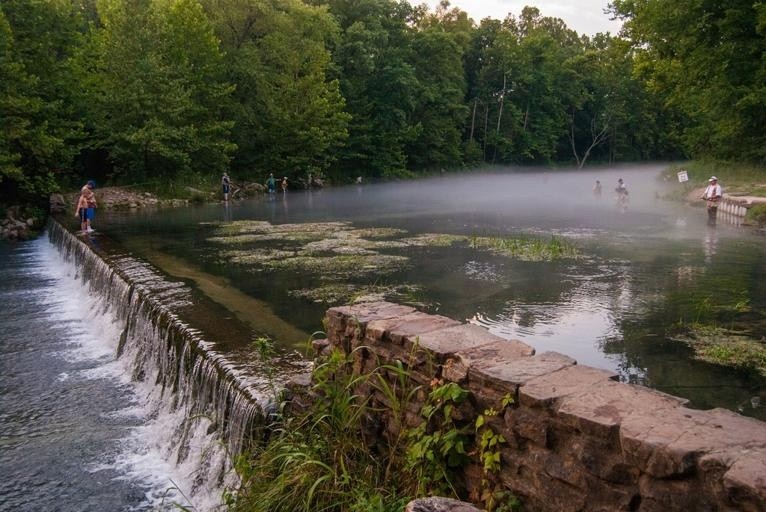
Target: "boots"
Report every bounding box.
[81,225,95,234]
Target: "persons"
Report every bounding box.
[265,173,282,202]
[591,179,603,207]
[615,178,630,213]
[222,172,230,202]
[81,180,97,234]
[74,189,89,237]
[699,175,722,228]
[280,176,290,200]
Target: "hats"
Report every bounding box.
[87,180,96,188]
[708,175,718,182]
